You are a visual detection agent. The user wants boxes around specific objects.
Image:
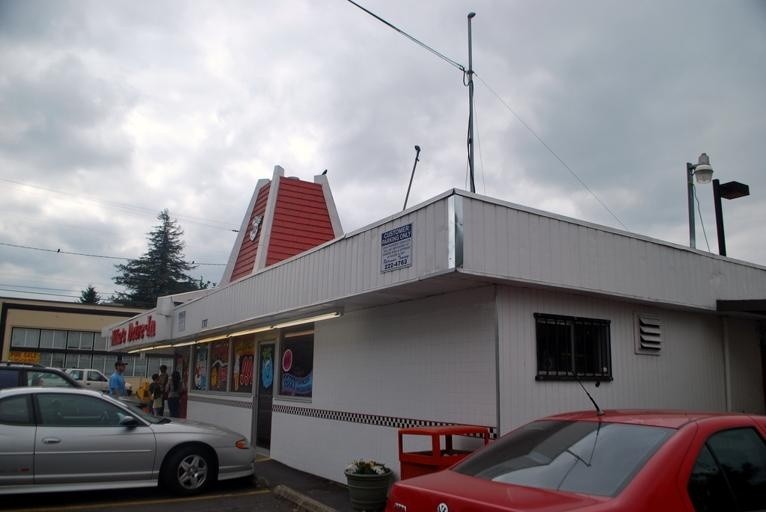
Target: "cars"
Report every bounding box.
[0,360,131,393]
[0,386,257,500]
[385,372,766,512]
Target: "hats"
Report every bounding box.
[114,360,128,366]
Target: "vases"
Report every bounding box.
[344,470,393,511]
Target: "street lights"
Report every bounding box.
[686,153,713,249]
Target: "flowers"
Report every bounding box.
[345,456,389,474]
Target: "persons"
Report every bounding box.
[108,361,129,396]
[147,365,182,418]
[30,376,43,386]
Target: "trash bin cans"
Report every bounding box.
[398,425,489,481]
